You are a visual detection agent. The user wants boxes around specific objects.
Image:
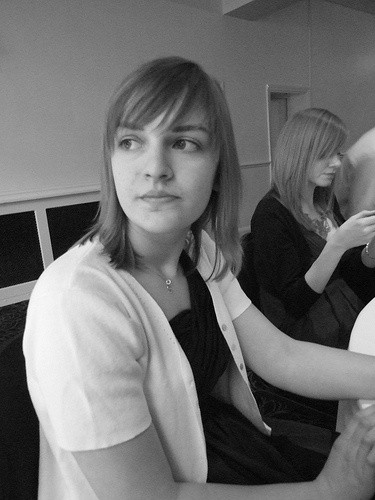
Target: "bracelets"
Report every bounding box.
[365,242,375,260]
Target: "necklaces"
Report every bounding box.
[137,259,183,292]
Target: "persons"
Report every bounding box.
[21,55,375,499]
[251,107,375,349]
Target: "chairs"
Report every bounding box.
[236,233,262,312]
[0,335,337,500]
